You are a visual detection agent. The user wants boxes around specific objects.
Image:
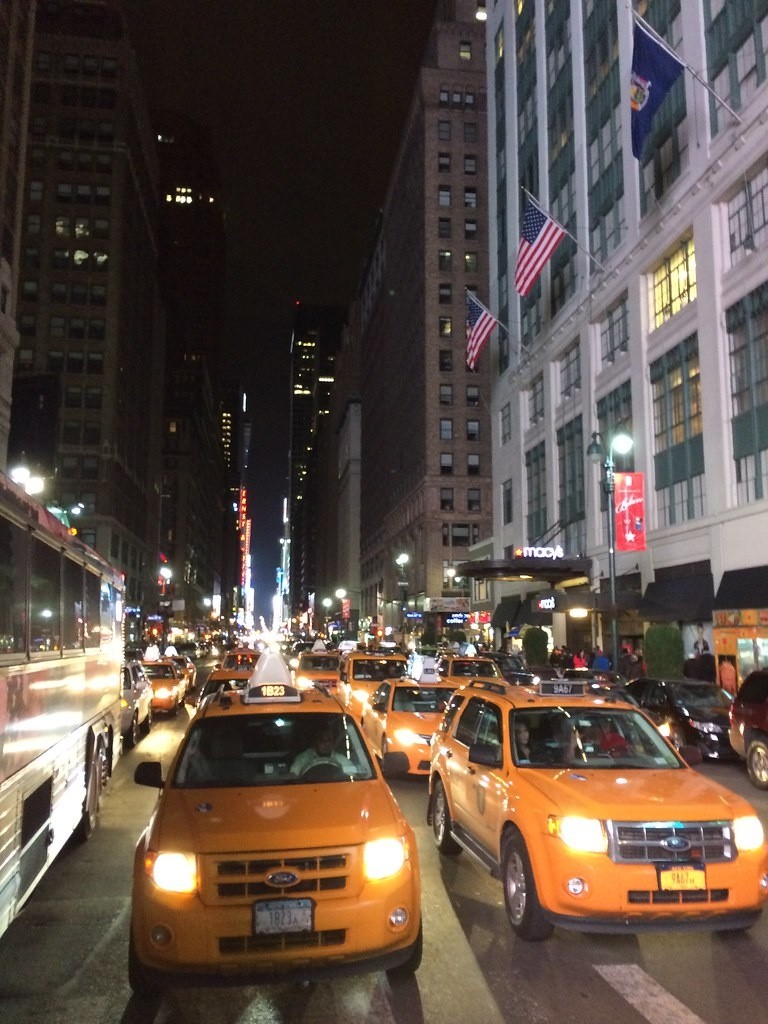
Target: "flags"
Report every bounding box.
[465,290,500,370]
[629,17,688,161]
[514,193,568,298]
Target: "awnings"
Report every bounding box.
[713,564,768,610]
[638,573,715,621]
[491,600,553,628]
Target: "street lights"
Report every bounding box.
[455,576,465,597]
[584,428,633,677]
[395,553,410,643]
[157,566,173,654]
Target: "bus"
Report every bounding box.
[0,467,127,941]
[128,649,424,999]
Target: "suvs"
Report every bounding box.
[290,638,314,653]
[425,675,768,938]
[727,664,768,792]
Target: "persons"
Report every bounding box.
[291,726,358,779]
[692,624,710,656]
[549,645,646,680]
[514,716,642,768]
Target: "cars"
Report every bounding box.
[119,645,198,751]
[202,647,534,769]
[624,677,735,761]
